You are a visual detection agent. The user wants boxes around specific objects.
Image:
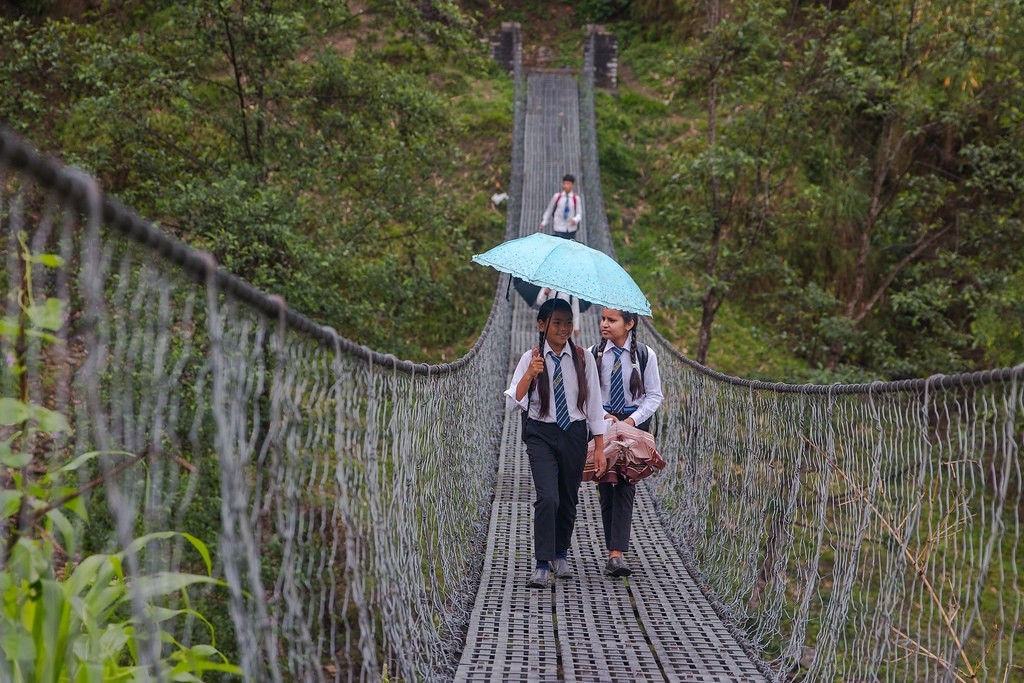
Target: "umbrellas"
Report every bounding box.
[513,277,592,313]
[472,232,653,357]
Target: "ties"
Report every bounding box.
[610,346,625,413]
[564,196,569,219]
[547,351,572,432]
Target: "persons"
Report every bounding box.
[587,306,664,578]
[540,174,581,239]
[536,287,580,338]
[503,298,612,589]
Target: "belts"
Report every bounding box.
[603,406,637,414]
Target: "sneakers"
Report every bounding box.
[603,557,632,577]
[527,565,550,589]
[548,558,572,578]
